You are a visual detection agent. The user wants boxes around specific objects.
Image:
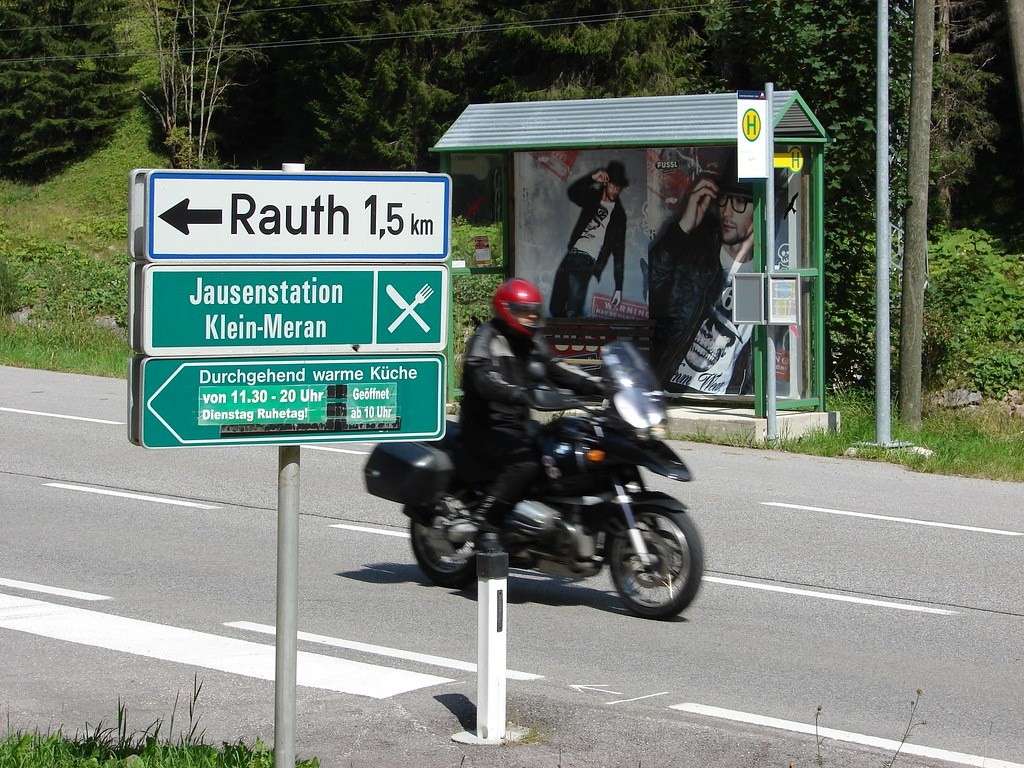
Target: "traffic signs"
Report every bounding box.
[136,168,452,450]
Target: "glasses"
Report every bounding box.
[609,181,623,191]
[713,193,753,213]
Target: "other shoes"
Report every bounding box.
[472,494,510,539]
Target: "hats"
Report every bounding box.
[605,162,631,186]
[712,158,754,201]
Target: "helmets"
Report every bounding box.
[493,277,545,340]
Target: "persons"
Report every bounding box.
[649,166,757,399]
[548,160,629,321]
[459,280,624,541]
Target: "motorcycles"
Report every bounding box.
[366,336,706,621]
